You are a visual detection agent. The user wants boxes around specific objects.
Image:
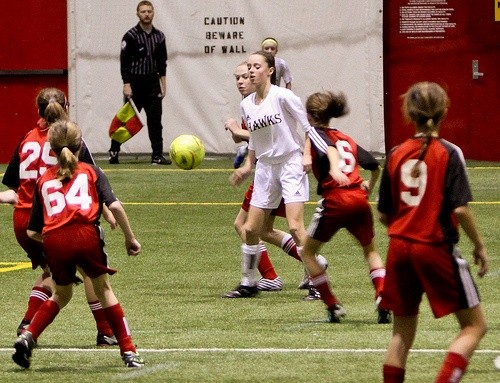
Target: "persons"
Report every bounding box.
[10,120,150,372]
[0,88,139,352]
[374,79,491,382]
[231,36,294,172]
[298,91,395,326]
[219,49,352,304]
[104,0,174,168]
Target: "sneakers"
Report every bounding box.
[325,305,347,322]
[121,350,145,367]
[17,319,30,336]
[97,334,121,348]
[305,284,322,300]
[233,151,244,168]
[298,255,328,290]
[152,154,171,165]
[225,284,258,298]
[12,331,35,368]
[257,276,282,290]
[109,149,119,163]
[378,311,392,323]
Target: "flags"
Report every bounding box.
[107,100,145,146]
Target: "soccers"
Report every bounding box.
[169,135,205,170]
